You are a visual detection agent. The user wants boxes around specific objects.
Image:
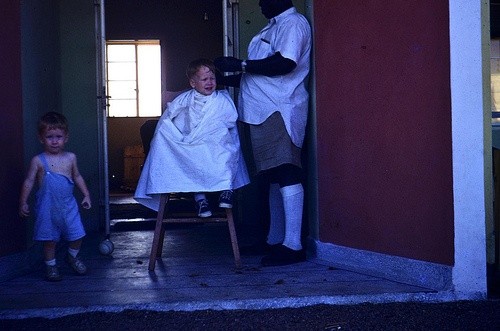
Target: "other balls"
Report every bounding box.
[99,239,115,256]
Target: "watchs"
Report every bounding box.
[241,59,247,75]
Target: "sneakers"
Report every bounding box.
[45,265,61,281]
[196,199,212,217]
[219,190,233,208]
[65,252,87,274]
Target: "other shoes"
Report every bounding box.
[263,244,306,266]
[249,241,283,254]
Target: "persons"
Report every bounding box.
[214,0,312,267]
[185,58,236,219]
[19,112,91,283]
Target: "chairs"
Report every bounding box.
[148,194,243,273]
